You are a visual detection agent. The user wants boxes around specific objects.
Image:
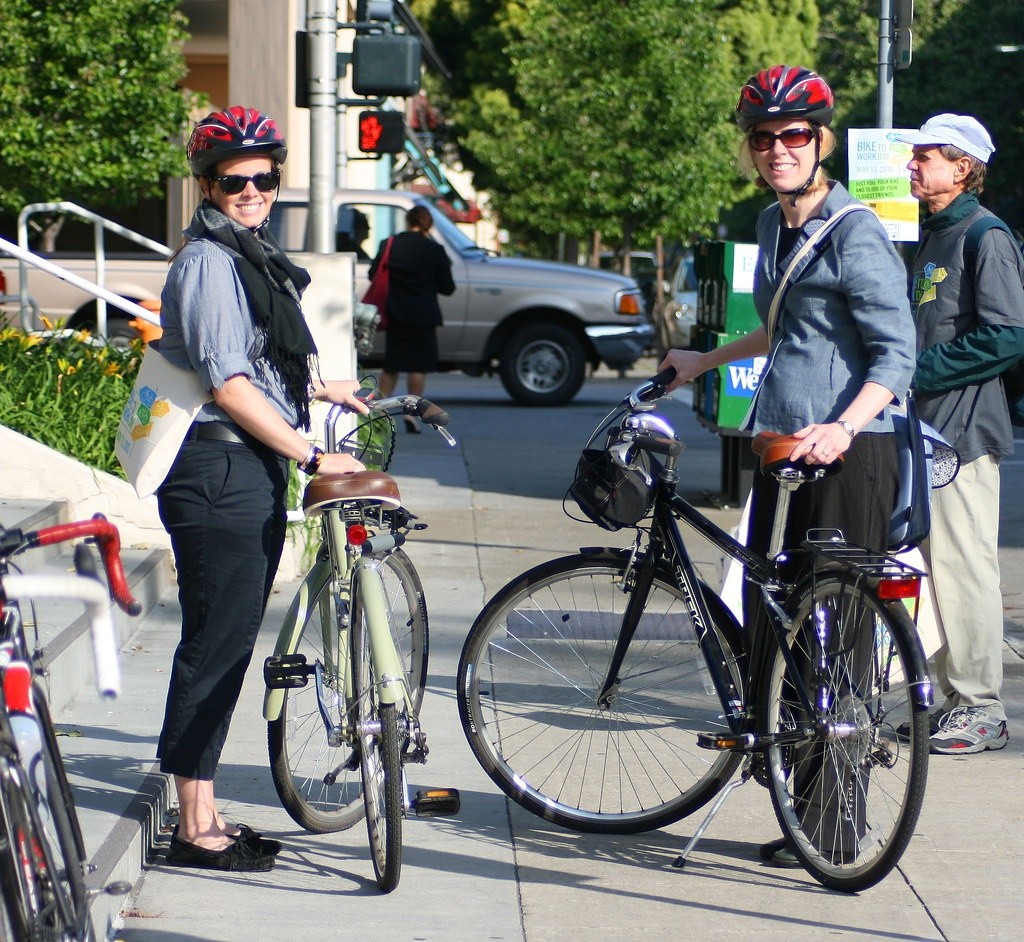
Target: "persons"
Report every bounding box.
[349,207,373,261]
[656,64,917,868]
[148,102,374,873]
[369,207,457,434]
[888,112,1024,756]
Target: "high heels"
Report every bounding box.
[404,414,421,433]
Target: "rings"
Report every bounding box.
[355,463,357,466]
[822,451,829,457]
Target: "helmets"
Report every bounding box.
[735,64,834,133]
[186,105,287,180]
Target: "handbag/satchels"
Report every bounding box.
[114,338,219,499]
[362,235,392,306]
[885,397,960,556]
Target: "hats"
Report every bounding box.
[896,114,996,165]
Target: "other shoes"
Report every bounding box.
[172,823,282,856]
[167,834,275,872]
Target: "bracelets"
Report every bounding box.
[299,442,325,476]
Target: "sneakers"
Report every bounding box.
[927,707,1009,755]
[895,708,951,741]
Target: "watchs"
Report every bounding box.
[835,421,855,439]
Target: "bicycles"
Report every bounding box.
[456,367,936,895]
[260,374,461,894]
[0,511,149,942]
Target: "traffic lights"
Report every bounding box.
[358,110,406,155]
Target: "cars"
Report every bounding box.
[652,249,700,364]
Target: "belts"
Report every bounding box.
[185,421,254,446]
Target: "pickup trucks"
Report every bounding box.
[0,189,657,408]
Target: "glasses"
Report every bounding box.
[206,169,279,194]
[748,128,817,151]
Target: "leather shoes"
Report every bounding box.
[773,845,857,868]
[761,836,788,859]
